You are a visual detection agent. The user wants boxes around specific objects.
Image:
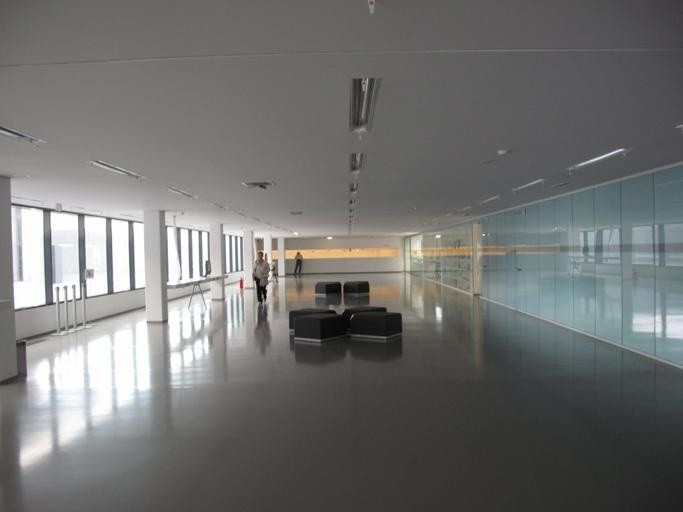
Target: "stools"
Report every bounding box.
[287,306,403,345]
[315,281,369,294]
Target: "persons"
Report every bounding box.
[293,251,303,275]
[269,260,278,282]
[252,252,270,303]
